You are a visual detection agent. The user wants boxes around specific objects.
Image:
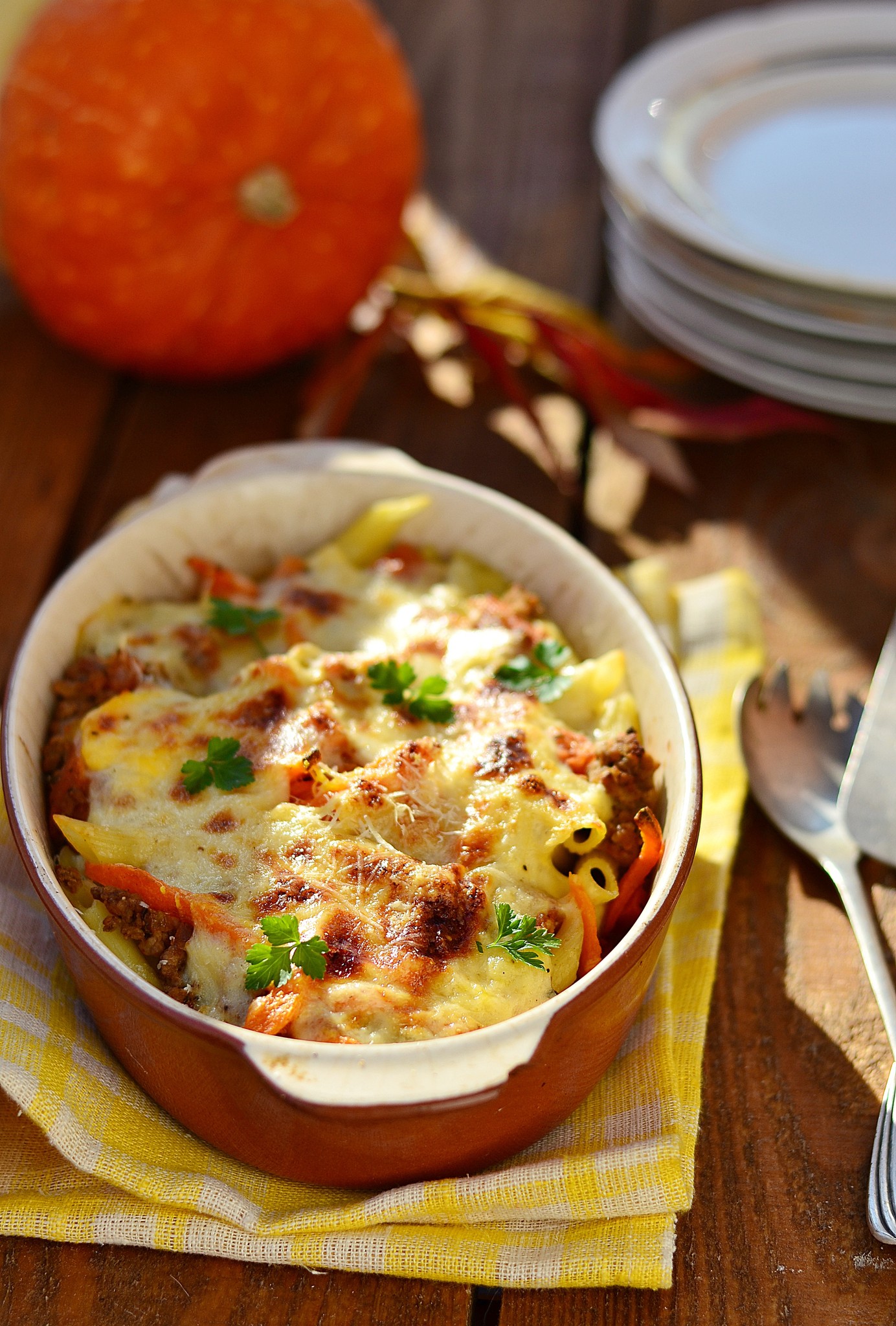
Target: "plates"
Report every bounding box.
[594,0,895,431]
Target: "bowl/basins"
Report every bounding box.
[0,441,706,1191]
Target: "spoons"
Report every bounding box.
[737,663,896,1057]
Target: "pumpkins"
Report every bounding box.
[0,0,432,377]
